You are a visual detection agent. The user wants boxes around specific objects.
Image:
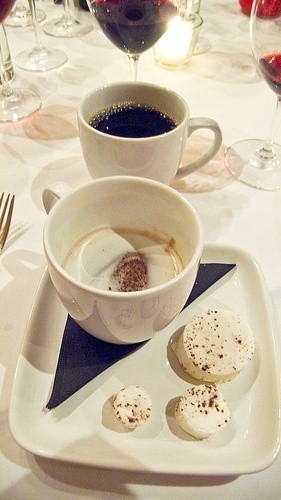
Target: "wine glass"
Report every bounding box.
[223,0,281,191]
[181,0,211,55]
[0,0,94,122]
[86,0,181,79]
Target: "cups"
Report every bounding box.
[43,176,203,344]
[239,0,281,16]
[76,80,222,187]
[151,9,204,68]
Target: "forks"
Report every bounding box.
[0,192,15,253]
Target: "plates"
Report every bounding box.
[7,242,281,476]
